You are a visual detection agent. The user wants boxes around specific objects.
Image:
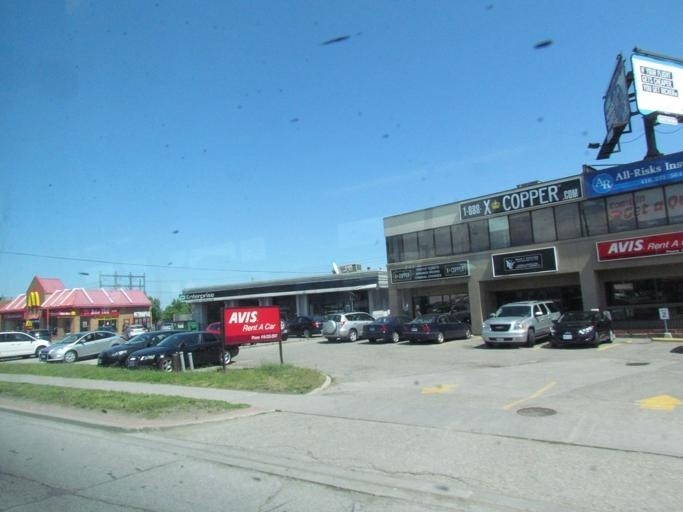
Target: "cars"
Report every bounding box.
[279,309,377,343]
[430,303,472,326]
[0,331,52,359]
[547,309,616,348]
[363,315,413,344]
[97,319,240,374]
[38,329,128,364]
[402,312,472,344]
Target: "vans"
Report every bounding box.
[479,298,563,347]
